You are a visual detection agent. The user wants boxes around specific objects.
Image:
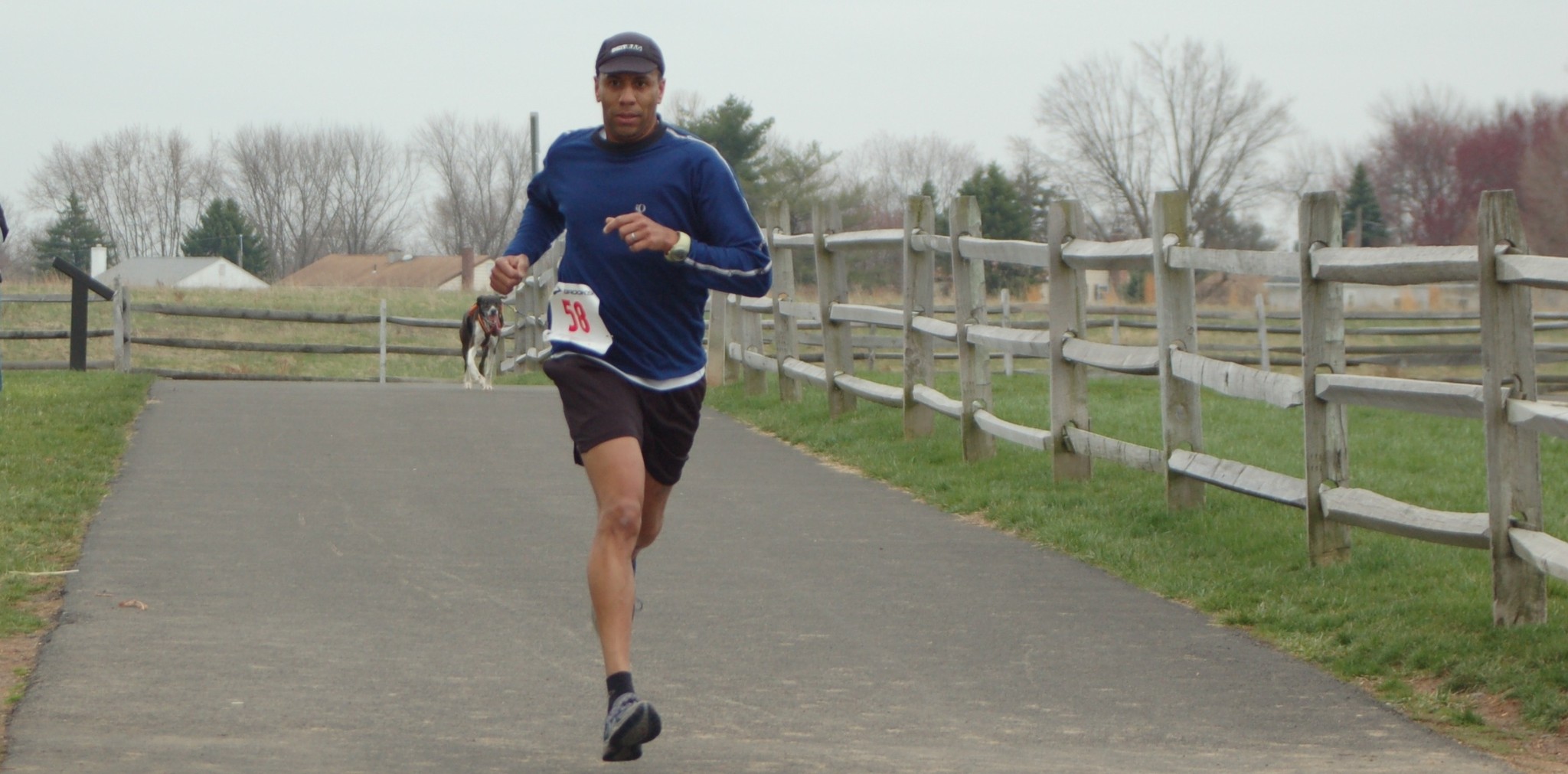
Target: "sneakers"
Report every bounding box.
[603,694,662,761]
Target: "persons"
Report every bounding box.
[487,34,774,761]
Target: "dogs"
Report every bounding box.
[461,295,505,390]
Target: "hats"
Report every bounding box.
[596,32,665,76]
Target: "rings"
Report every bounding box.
[631,232,636,242]
